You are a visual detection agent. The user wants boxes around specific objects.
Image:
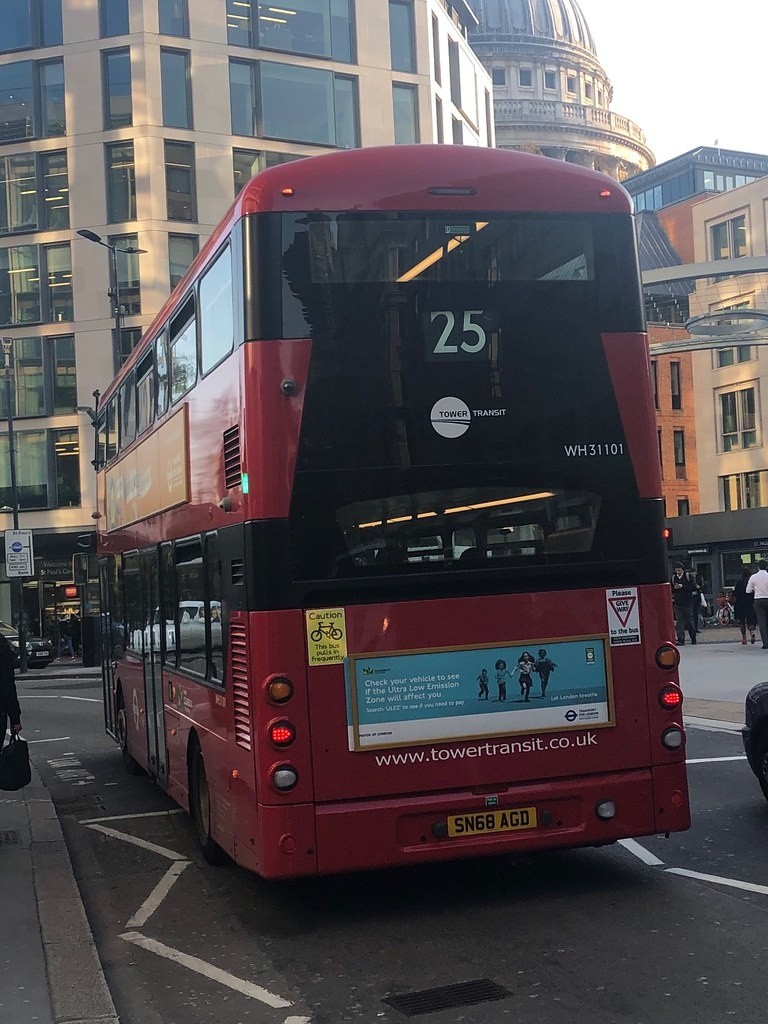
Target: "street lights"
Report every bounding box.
[74,389,105,615]
[76,226,149,379]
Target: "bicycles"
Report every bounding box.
[717,588,736,626]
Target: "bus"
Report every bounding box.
[94,144,691,882]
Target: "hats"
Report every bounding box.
[690,567,696,573]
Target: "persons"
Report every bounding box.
[734,560,768,649]
[670,562,701,646]
[0,632,21,753]
[54,628,76,662]
[476,649,558,702]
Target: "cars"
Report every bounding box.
[0,619,57,669]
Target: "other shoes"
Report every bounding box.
[676,641,685,646]
[691,637,696,644]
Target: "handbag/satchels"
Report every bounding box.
[700,593,707,607]
[0,733,31,793]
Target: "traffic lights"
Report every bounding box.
[64,583,79,598]
[664,528,674,551]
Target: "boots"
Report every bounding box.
[750,630,755,645]
[742,634,747,644]
